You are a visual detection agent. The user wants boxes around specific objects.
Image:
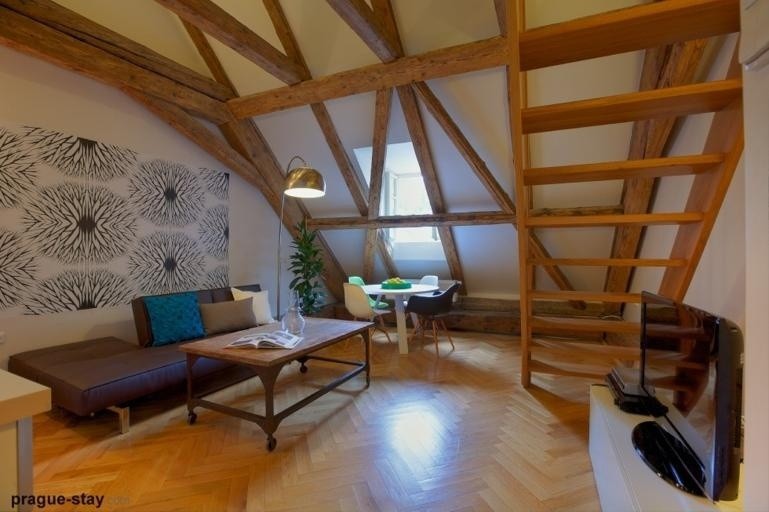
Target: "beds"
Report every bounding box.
[10,282,273,436]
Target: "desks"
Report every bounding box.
[1,367,52,512]
[588,385,744,512]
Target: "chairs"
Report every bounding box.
[343,275,459,357]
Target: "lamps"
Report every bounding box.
[275,155,326,321]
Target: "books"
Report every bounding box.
[224,331,303,350]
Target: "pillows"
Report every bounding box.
[142,286,275,347]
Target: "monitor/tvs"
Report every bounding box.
[631,290,744,503]
